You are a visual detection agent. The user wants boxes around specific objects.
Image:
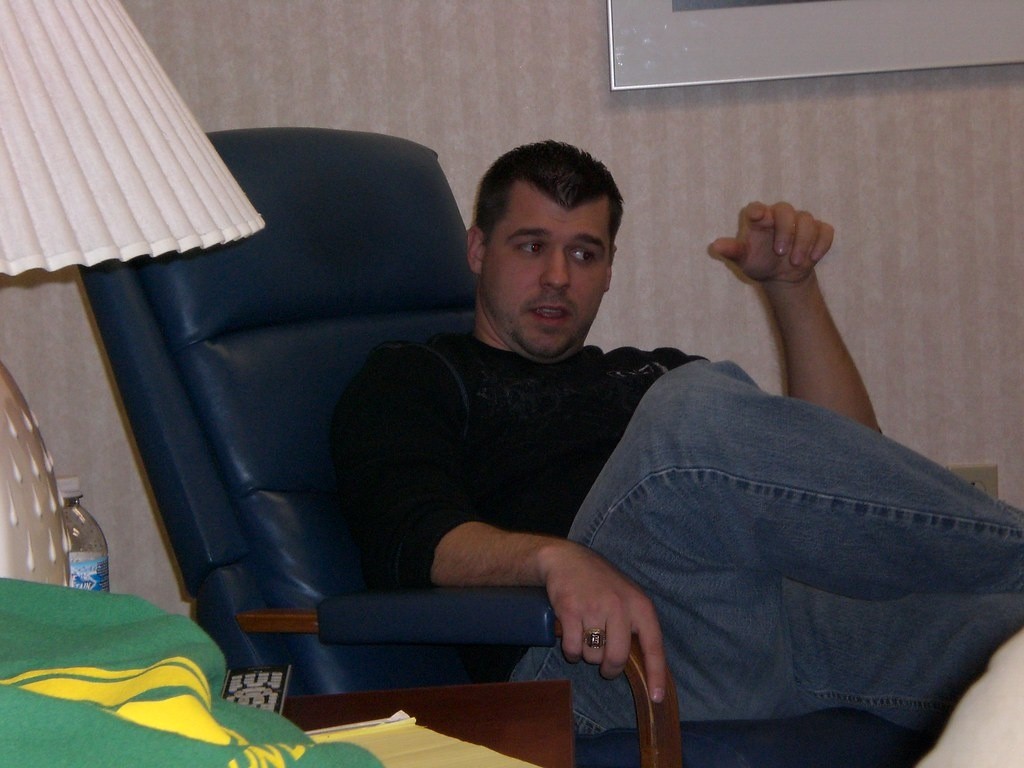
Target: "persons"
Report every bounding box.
[328,140,1024,737]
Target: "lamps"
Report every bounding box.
[0,0,267,586]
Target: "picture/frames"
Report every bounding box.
[606,0,1024,92]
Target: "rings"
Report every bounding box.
[583,628,606,648]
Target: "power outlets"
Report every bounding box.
[951,464,999,499]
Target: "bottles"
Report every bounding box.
[57,476,110,593]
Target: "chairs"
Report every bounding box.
[76,129,939,768]
